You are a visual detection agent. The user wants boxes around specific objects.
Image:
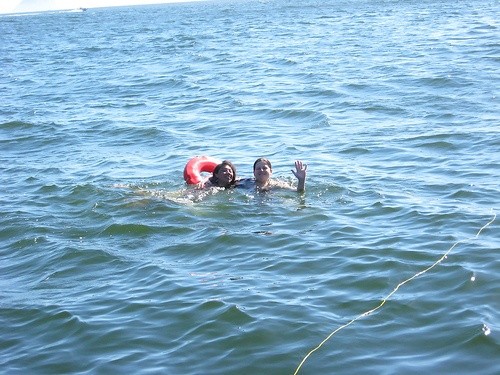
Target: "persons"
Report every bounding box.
[195,156,308,197]
[203,161,236,189]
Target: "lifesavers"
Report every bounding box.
[182,152,222,188]
